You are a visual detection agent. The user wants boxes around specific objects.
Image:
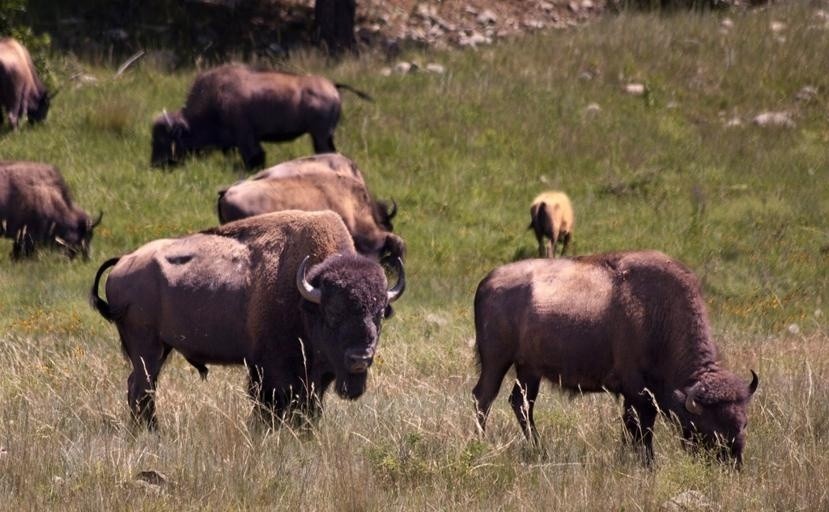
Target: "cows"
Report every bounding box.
[217,151,405,257]
[151,63,376,172]
[88,209,406,441]
[471,249,758,471]
[527,190,574,258]
[0,160,103,262]
[0,36,63,133]
